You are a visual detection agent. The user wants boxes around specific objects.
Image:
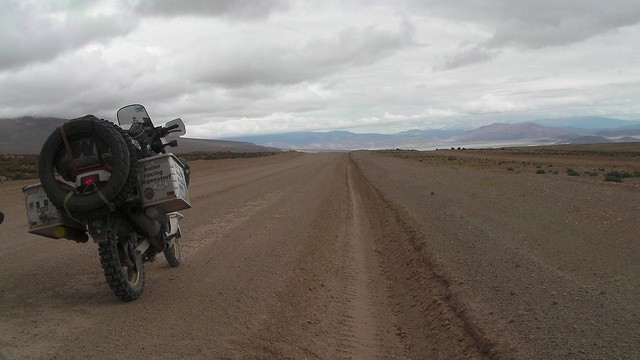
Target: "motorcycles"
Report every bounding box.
[21,103,194,303]
